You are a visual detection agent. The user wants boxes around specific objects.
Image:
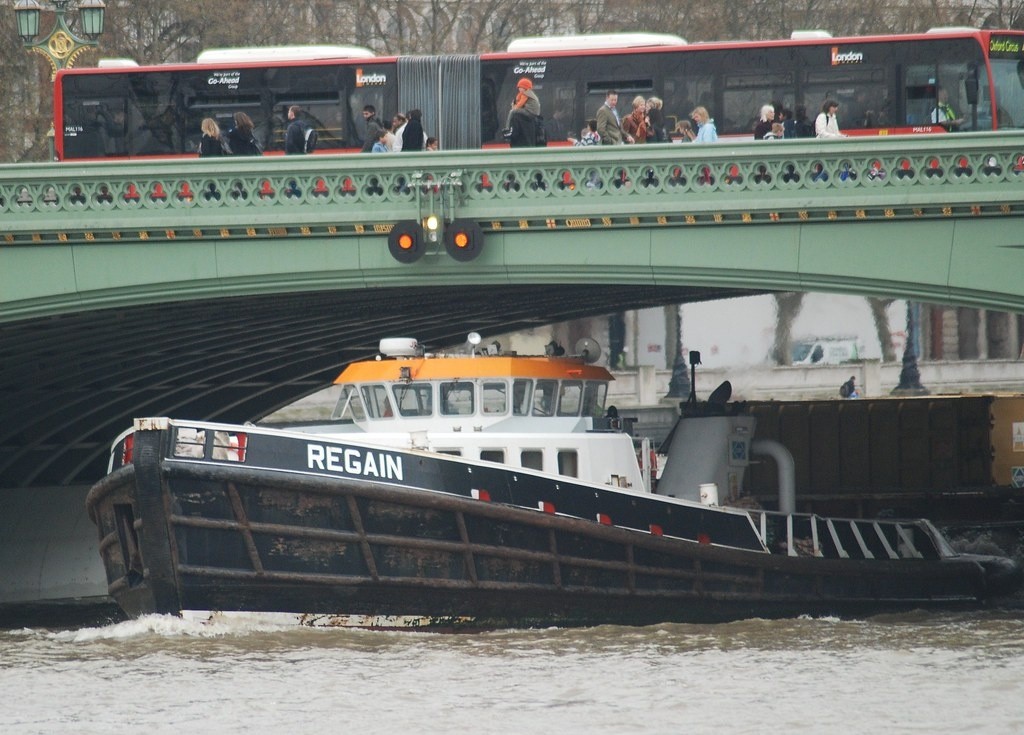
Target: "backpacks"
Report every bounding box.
[300,126,319,154]
[809,111,829,137]
[840,385,851,398]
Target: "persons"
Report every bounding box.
[227,112,262,200]
[284,105,318,198]
[567,90,673,189]
[544,104,576,141]
[754,104,812,185]
[839,376,859,398]
[675,107,717,186]
[198,118,232,201]
[931,88,969,132]
[501,78,548,149]
[360,105,438,195]
[812,101,848,183]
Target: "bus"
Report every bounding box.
[53,25,1024,200]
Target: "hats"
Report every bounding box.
[516,78,533,90]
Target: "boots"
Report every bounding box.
[503,126,514,137]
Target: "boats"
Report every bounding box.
[86,333,1024,623]
